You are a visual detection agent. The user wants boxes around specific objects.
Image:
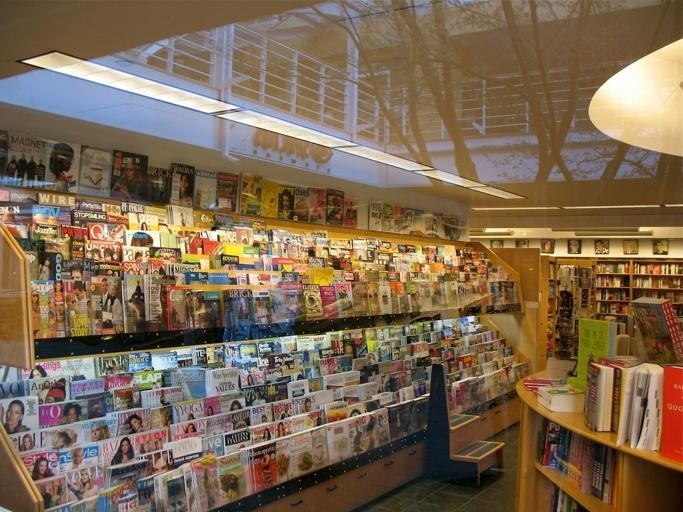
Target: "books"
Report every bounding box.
[1,128,474,511]
[474,249,683,512]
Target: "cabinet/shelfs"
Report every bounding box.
[513,369,683,511]
[546,256,682,362]
